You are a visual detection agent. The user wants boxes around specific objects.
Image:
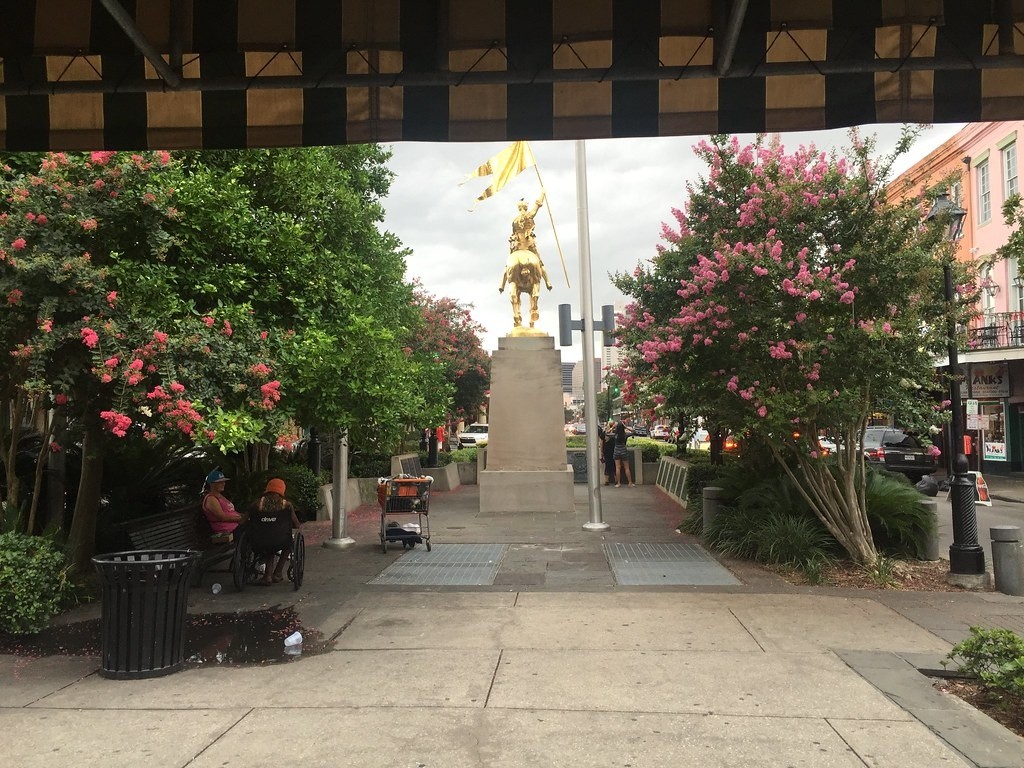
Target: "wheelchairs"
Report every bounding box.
[232,510,306,592]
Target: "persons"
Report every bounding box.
[201,470,301,585]
[419,419,462,454]
[598,420,635,488]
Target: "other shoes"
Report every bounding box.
[259,577,272,585]
[615,484,621,488]
[272,573,283,582]
[628,483,635,487]
[605,482,609,486]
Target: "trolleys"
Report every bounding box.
[378,477,435,554]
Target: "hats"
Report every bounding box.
[207,471,230,483]
[264,478,286,496]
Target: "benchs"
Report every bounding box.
[120,502,238,590]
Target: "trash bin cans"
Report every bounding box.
[90,550,199,681]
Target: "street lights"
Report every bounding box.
[921,191,967,356]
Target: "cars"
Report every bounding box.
[455,422,489,448]
[565,420,587,434]
[650,424,669,440]
[828,426,937,483]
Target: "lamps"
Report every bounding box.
[921,186,969,256]
